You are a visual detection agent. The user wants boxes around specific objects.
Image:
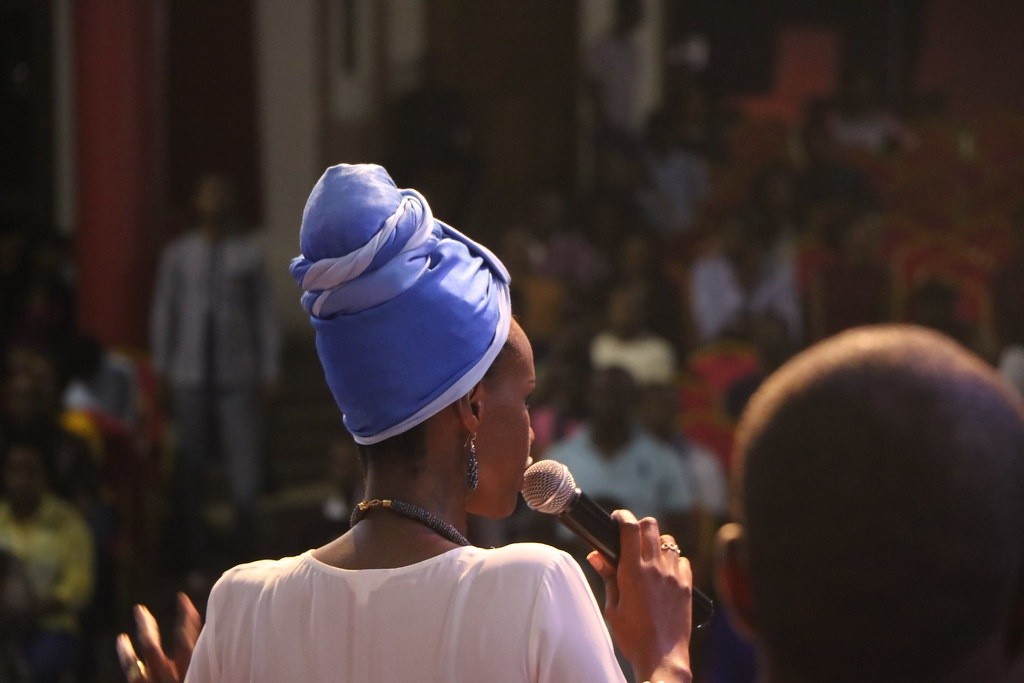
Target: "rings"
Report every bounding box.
[126,660,150,683]
[661,542,681,556]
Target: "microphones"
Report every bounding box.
[521,460,716,627]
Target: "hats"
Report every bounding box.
[289,163,511,445]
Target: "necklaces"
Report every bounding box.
[349,497,472,546]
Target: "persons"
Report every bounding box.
[112,0,1021,683]
[0,219,145,682]
